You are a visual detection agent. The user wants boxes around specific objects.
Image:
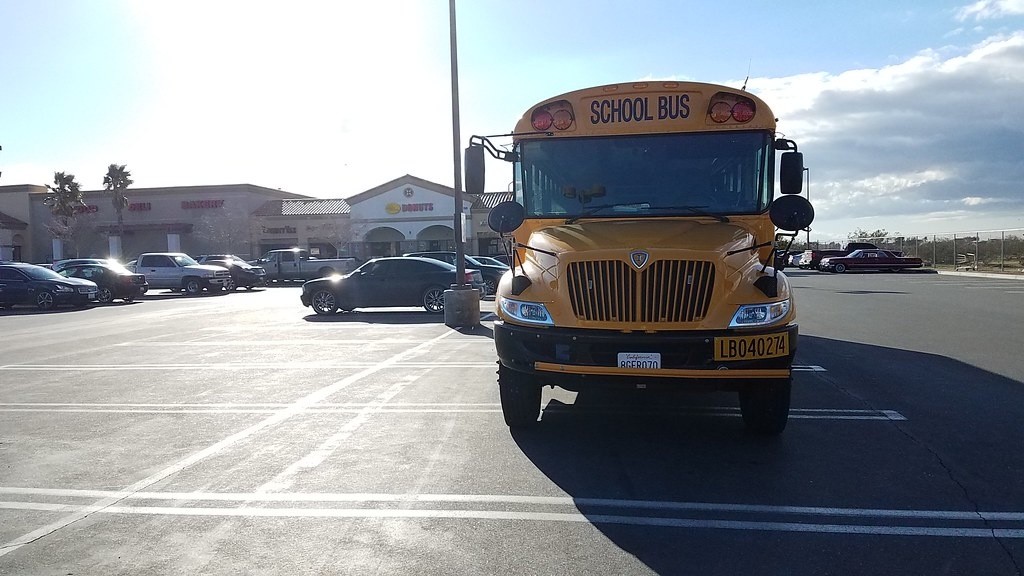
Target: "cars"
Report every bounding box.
[777,242,924,274]
[300,257,487,316]
[0,265,99,311]
[0,259,148,305]
[403,251,513,295]
[194,254,267,291]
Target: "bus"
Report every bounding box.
[464,82,815,441]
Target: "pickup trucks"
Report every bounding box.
[246,249,356,285]
[122,252,231,296]
